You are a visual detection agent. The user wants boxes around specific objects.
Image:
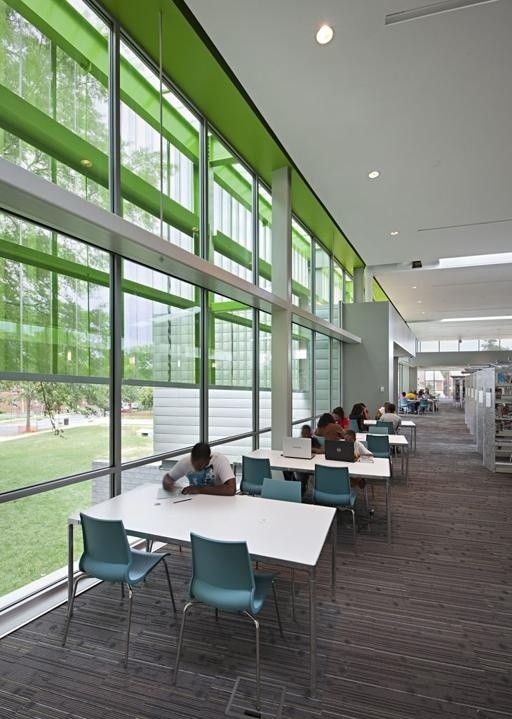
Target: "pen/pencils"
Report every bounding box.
[173,499,192,504]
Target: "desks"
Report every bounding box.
[66,482,339,699]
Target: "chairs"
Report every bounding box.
[238,420,421,544]
[172,531,283,711]
[398,394,439,416]
[255,476,302,623]
[60,512,177,669]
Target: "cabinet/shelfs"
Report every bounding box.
[451,365,511,474]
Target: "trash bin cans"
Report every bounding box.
[65,418,69,425]
[456,402,460,409]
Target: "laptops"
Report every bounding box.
[324,439,358,462]
[282,437,315,460]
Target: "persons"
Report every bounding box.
[332,407,350,433]
[379,401,396,417]
[283,425,324,496]
[380,403,400,453]
[315,413,345,441]
[357,403,369,420]
[344,429,373,489]
[163,442,236,497]
[349,404,368,431]
[400,388,437,413]
